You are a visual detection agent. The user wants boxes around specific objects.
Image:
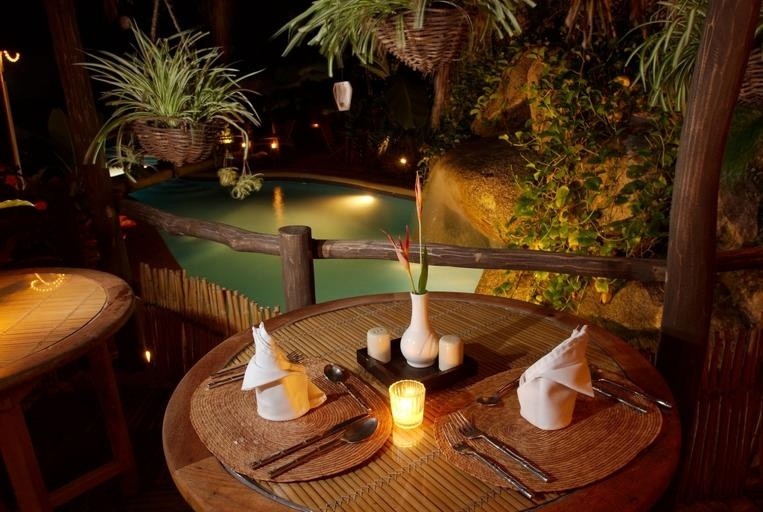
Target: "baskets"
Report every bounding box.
[737,48,763,104]
[133,115,225,166]
[367,3,473,74]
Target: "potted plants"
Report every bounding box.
[67,17,266,202]
[615,0,763,114]
[270,0,539,78]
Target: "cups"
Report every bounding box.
[438,335,464,373]
[388,375,426,428]
[363,325,391,365]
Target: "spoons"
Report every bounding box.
[262,417,378,480]
[323,362,372,411]
[476,373,520,408]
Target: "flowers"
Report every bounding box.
[378,172,431,294]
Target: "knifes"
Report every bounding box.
[247,408,369,471]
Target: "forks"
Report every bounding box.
[448,408,556,483]
[584,360,674,409]
[207,348,303,389]
[442,422,546,500]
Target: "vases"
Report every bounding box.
[399,293,440,369]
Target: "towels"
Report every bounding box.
[516,324,595,431]
[240,321,327,421]
